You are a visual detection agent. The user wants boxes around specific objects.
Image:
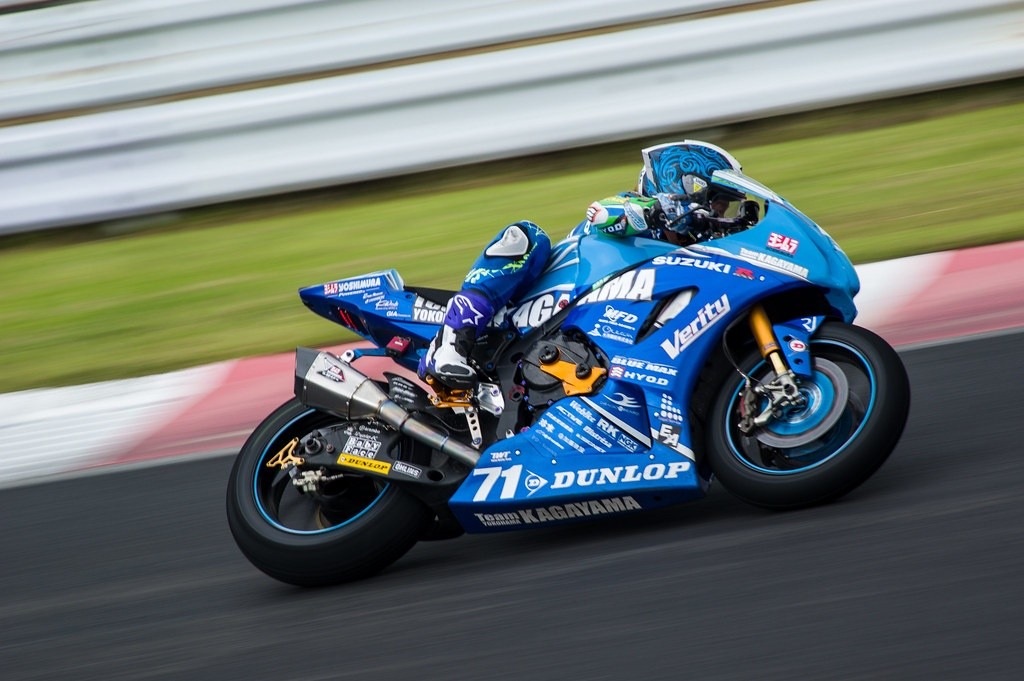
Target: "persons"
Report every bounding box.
[417,138,750,385]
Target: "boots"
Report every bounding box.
[415,289,497,387]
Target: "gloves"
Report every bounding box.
[648,190,717,239]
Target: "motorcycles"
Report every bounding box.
[224,168,912,589]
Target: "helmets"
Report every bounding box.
[635,139,746,200]
